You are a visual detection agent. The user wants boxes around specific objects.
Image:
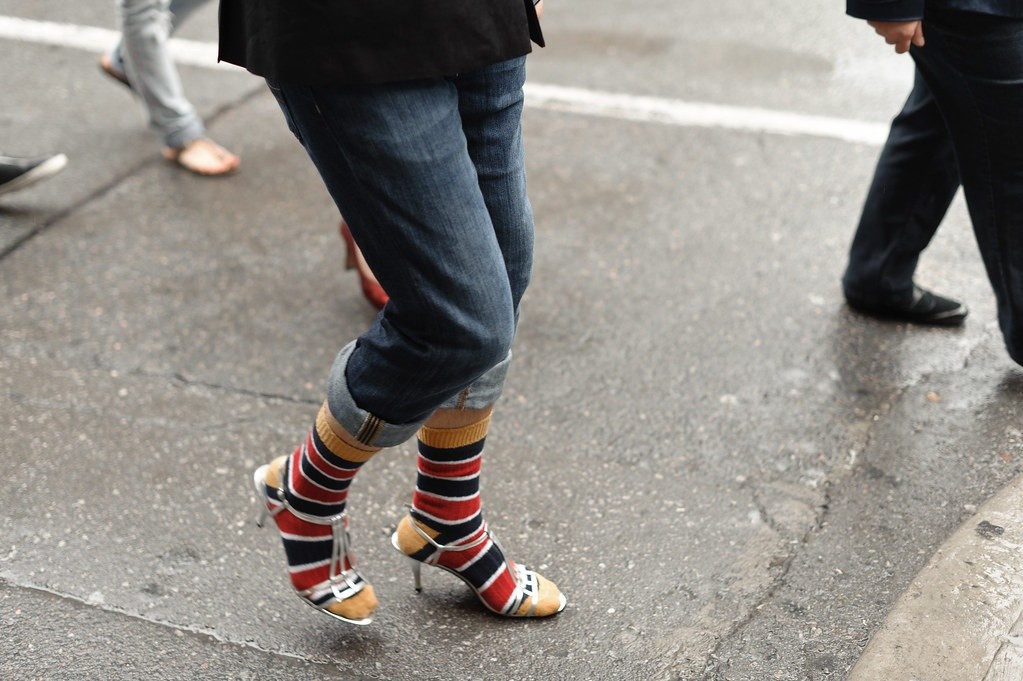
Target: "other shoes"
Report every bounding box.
[0,151,68,195]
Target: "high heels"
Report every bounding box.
[253,457,375,626]
[391,503,566,617]
[338,218,389,308]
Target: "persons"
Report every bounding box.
[97,0,243,177]
[0,149,68,195]
[215,1,568,625]
[340,221,386,311]
[845,0,1023,367]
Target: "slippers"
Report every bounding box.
[99,56,134,87]
[161,136,239,176]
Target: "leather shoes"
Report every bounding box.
[842,273,968,325]
